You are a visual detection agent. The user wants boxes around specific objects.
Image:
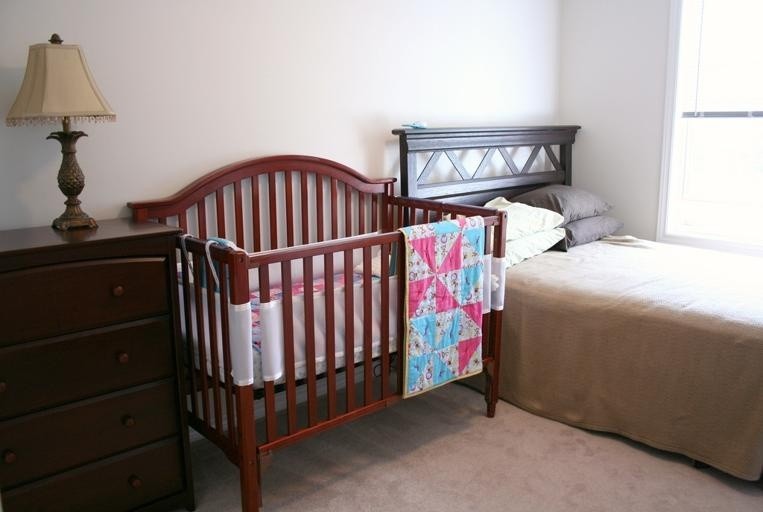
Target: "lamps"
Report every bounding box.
[6,36,116,229]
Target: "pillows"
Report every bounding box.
[558,218,622,252]
[483,196,563,242]
[490,227,565,266]
[515,184,611,224]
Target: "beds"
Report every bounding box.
[395,122,763,483]
[127,155,507,512]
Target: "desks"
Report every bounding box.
[0,208,185,511]
